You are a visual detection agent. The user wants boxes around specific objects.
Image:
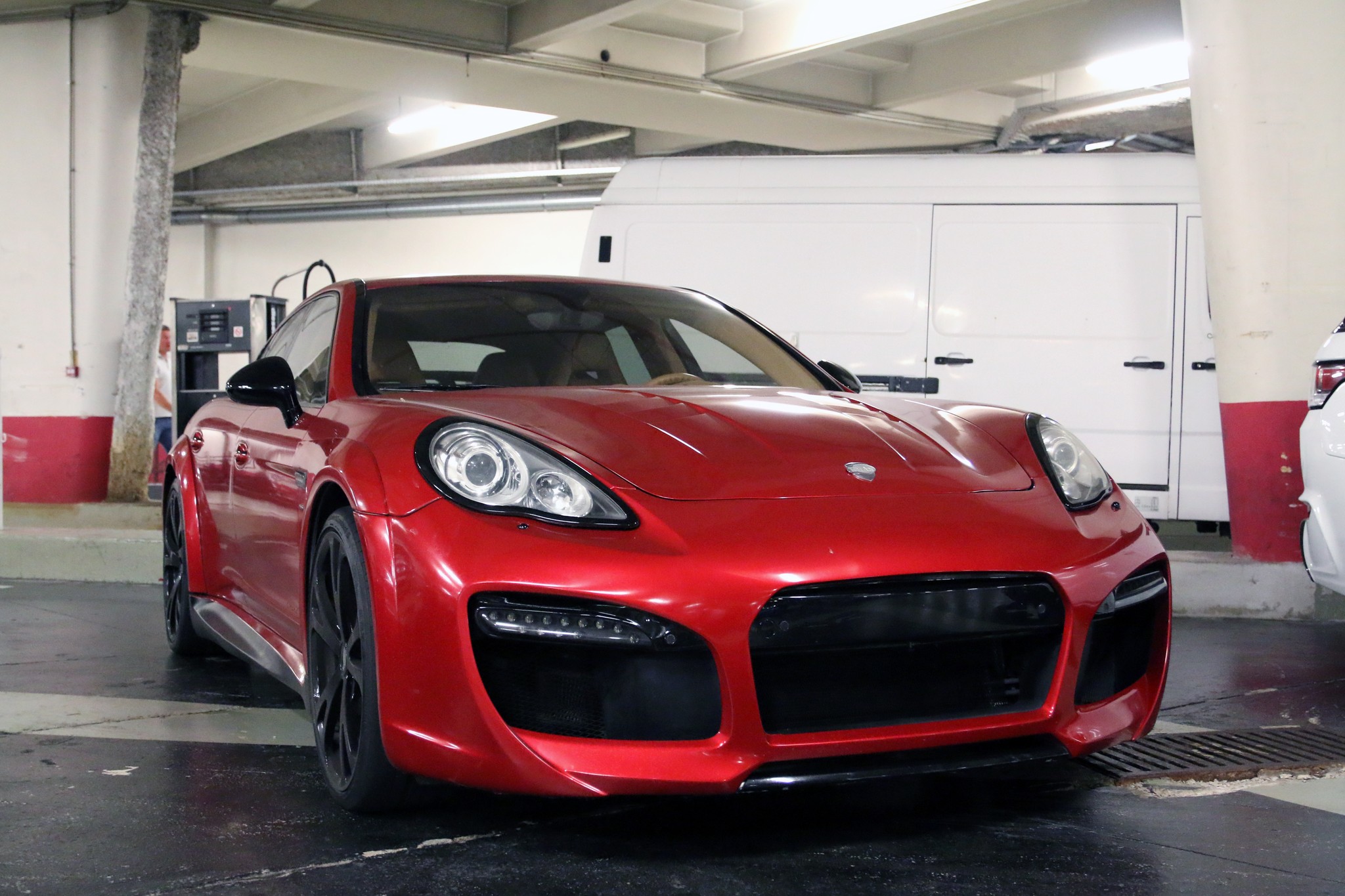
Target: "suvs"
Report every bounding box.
[1299,317,1345,591]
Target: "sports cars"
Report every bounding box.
[159,274,1177,809]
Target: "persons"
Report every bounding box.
[153,327,173,454]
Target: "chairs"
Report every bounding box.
[312,331,429,389]
[500,328,627,388]
